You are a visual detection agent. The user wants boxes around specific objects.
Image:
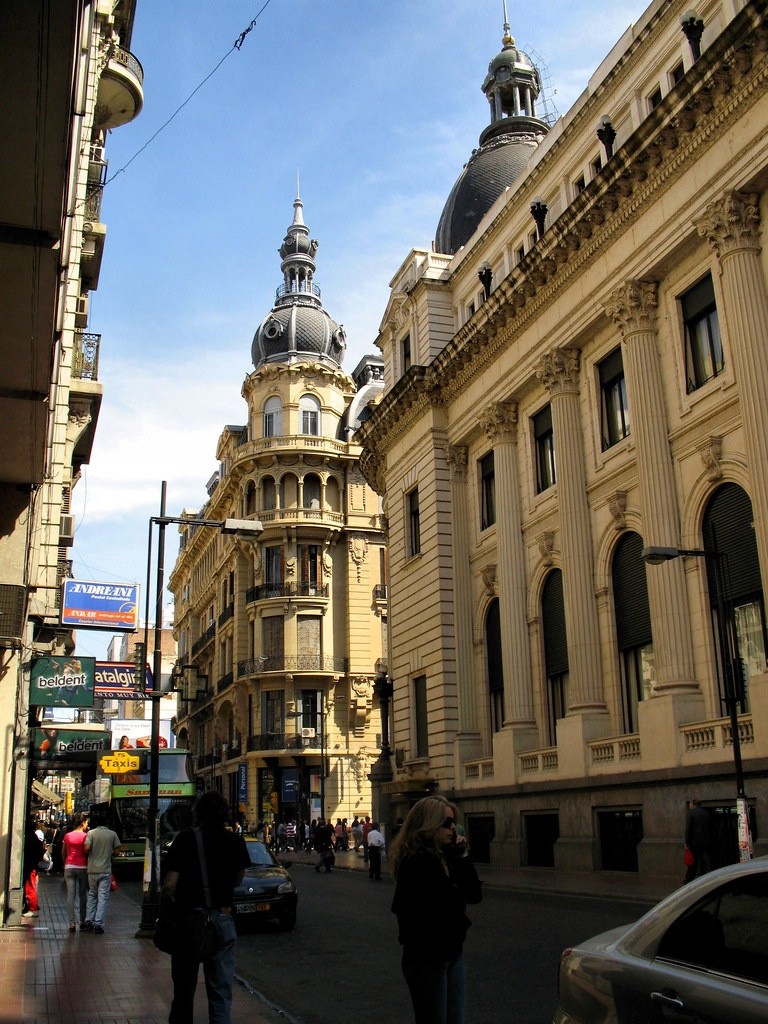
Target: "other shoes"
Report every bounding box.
[313,866,322,873]
[85,921,94,931]
[325,867,333,872]
[22,910,39,917]
[95,925,105,934]
[78,920,85,931]
[69,923,76,930]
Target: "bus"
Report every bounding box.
[77,749,194,881]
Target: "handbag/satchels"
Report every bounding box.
[47,843,52,854]
[153,912,227,956]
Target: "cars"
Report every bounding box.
[563,853,768,1024]
[229,838,297,933]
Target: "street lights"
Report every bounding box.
[135,480,265,938]
[639,543,755,863]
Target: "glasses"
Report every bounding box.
[441,816,455,830]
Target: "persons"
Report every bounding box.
[314,821,336,872]
[391,817,404,877]
[155,791,252,1024]
[389,795,484,1024]
[82,812,122,935]
[367,821,387,881]
[683,797,712,886]
[363,815,373,863]
[120,736,133,750]
[234,816,365,852]
[22,808,84,917]
[61,812,90,932]
